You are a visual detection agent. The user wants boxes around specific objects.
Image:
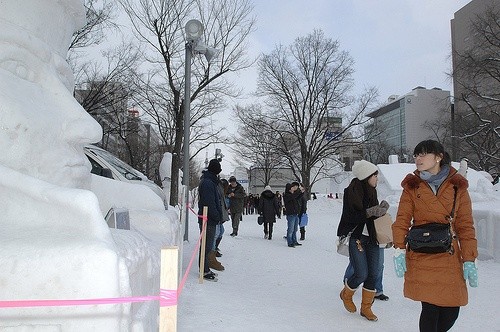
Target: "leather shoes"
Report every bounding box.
[375,293,389,301]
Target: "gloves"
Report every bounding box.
[367,205,387,217]
[463,261,478,287]
[393,248,407,277]
[381,200,390,210]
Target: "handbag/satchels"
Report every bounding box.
[336,244,350,257]
[258,216,264,225]
[375,213,394,245]
[300,213,308,227]
[405,222,451,253]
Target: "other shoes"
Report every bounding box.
[203,269,218,282]
[289,242,303,248]
[230,232,237,236]
[264,233,272,240]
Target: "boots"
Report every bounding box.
[300,229,306,240]
[215,236,223,257]
[208,251,225,271]
[340,279,356,313]
[361,287,378,320]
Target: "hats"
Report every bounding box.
[352,159,378,180]
[208,159,222,174]
[264,186,271,190]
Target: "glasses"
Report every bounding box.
[414,152,434,159]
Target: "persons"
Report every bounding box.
[198,158,245,282]
[337,160,389,320]
[391,140,479,332]
[245,181,308,247]
